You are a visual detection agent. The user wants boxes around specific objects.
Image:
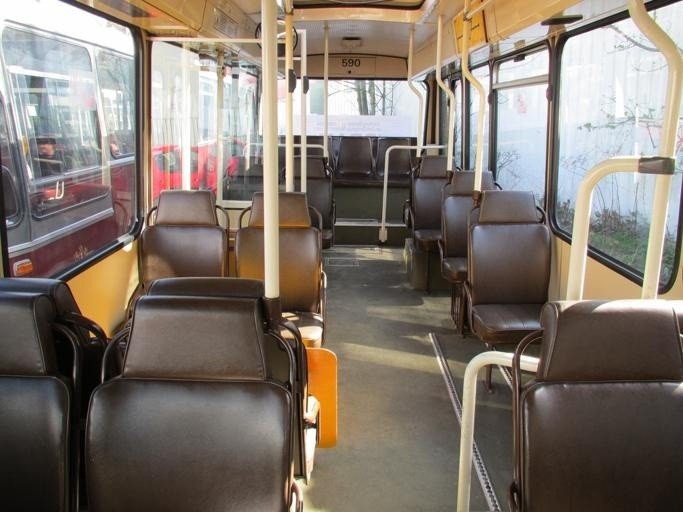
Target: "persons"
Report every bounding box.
[37,138,63,176]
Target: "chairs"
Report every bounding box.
[220,135,330,203]
[333,135,414,187]
[0,278,333,512]
[508,299,683,512]
[465,191,552,388]
[439,166,502,334]
[124,189,231,326]
[408,157,463,295]
[279,157,335,253]
[235,192,326,343]
